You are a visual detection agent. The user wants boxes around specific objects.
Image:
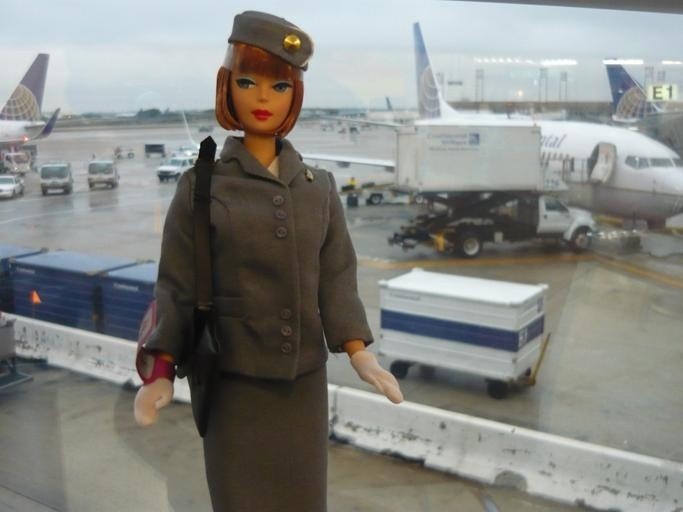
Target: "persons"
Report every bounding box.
[131,10,404,512]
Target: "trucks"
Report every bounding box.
[88,161,119,188]
[1,152,31,176]
[39,163,73,193]
[387,124,596,258]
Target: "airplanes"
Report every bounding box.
[297,22,683,219]
[0,53,60,147]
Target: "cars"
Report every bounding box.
[0,174,25,199]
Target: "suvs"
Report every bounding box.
[158,157,195,181]
